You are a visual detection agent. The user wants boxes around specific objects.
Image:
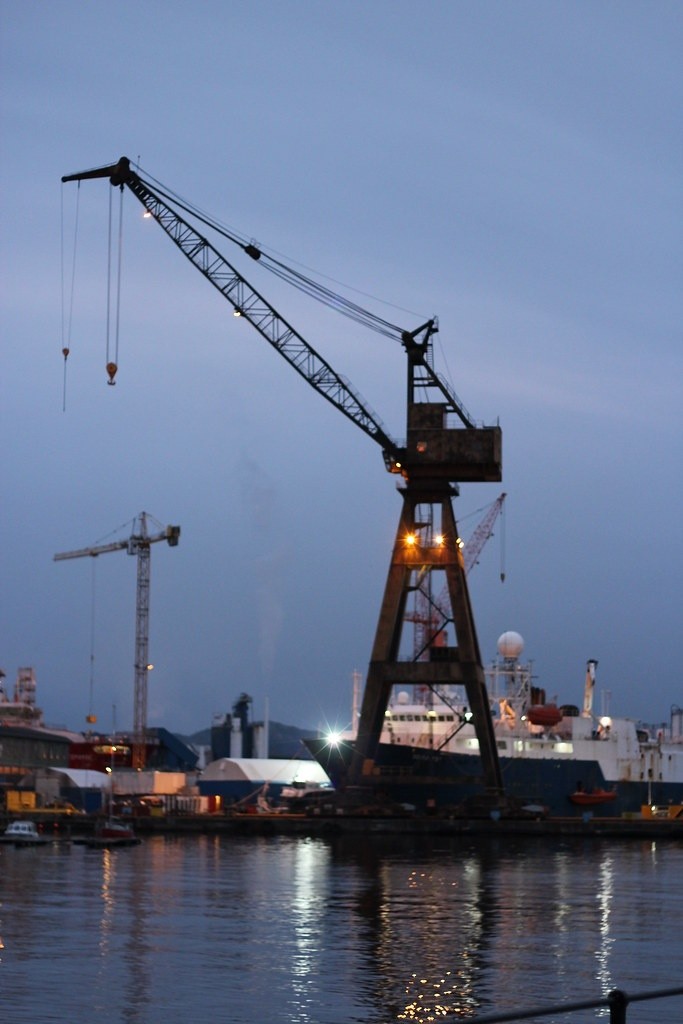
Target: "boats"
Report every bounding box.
[101,821,134,838]
[5,820,39,838]
[300,660,683,821]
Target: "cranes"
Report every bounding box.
[53,511,181,770]
[56,158,510,800]
